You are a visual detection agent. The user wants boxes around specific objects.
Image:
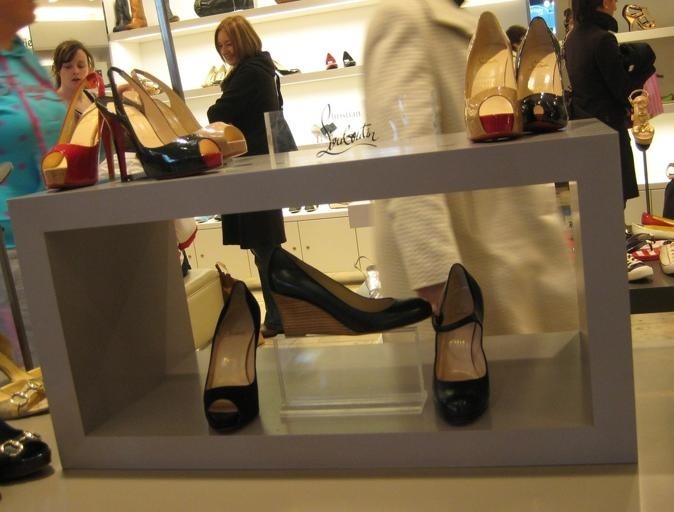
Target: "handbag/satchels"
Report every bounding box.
[195,0,254,17]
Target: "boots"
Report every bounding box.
[126,0,147,29]
[163,0,180,21]
[113,0,131,32]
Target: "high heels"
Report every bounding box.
[432,263,490,428]
[266,246,434,337]
[203,280,260,434]
[515,17,569,131]
[42,67,248,188]
[463,11,522,142]
[624,3,657,32]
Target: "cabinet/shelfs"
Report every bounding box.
[7,118,639,470]
[100,0,377,289]
[18,0,114,97]
[556,0,674,225]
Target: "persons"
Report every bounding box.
[363,1,576,344]
[50,40,98,116]
[505,25,523,69]
[0,0,73,367]
[563,0,640,200]
[207,15,286,337]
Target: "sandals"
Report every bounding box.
[0,353,49,419]
[0,418,52,483]
[629,89,654,145]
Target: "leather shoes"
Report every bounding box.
[326,53,337,69]
[343,51,356,66]
[273,60,299,74]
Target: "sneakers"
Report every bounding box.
[625,212,674,281]
